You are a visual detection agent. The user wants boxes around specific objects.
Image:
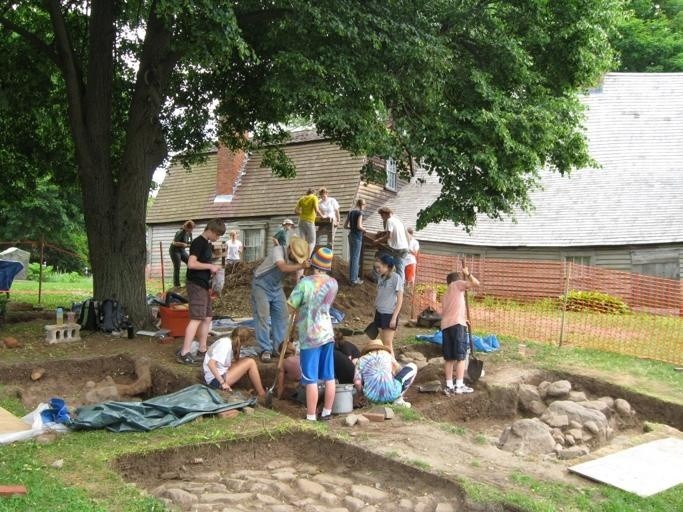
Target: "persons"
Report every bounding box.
[250,238,311,363]
[374,255,404,357]
[374,207,418,289]
[176,218,226,367]
[202,327,266,397]
[272,188,339,260]
[222,231,243,264]
[287,248,339,419]
[343,198,367,286]
[169,221,195,289]
[441,267,480,394]
[276,328,417,408]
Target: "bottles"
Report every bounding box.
[220,240,228,257]
[127,324,134,340]
[56,307,64,327]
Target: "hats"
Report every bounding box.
[282,220,294,226]
[309,247,333,271]
[361,339,391,354]
[290,235,310,264]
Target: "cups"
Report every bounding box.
[66,312,76,325]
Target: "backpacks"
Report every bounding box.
[100,299,124,333]
[78,299,100,329]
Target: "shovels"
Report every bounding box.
[264,275,305,410]
[462,259,483,381]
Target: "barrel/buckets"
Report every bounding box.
[331,383,354,414]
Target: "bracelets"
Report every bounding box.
[467,273,470,279]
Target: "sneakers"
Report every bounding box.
[318,412,331,420]
[351,277,363,285]
[197,351,206,357]
[455,385,474,394]
[261,352,270,362]
[176,352,200,367]
[443,387,455,397]
[393,398,411,409]
